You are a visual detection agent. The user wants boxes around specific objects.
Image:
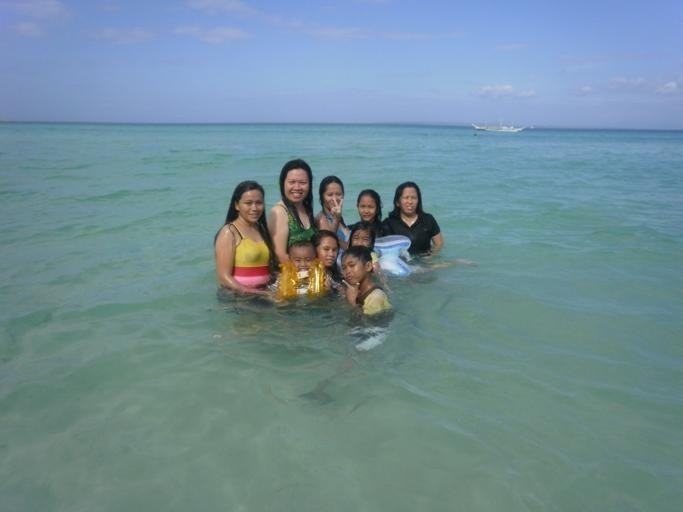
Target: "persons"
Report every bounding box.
[276,241,320,296]
[310,229,339,284]
[345,222,389,291]
[346,189,383,243]
[317,175,352,269]
[338,248,393,351]
[269,160,317,276]
[213,180,274,303]
[383,182,441,268]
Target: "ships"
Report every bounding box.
[467,119,527,133]
